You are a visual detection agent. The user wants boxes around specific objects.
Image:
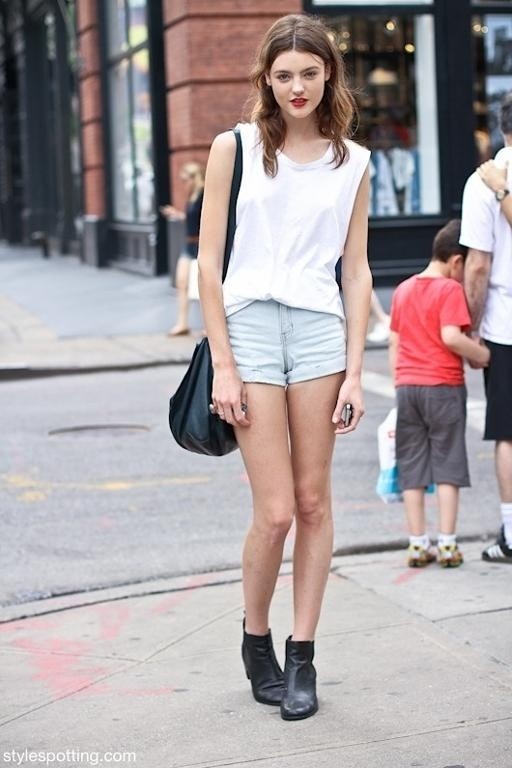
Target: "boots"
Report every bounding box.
[281,635,318,720]
[242,618,284,705]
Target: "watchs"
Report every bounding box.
[496,190,508,201]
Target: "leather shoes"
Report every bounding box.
[166,329,190,337]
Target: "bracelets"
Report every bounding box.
[469,330,480,337]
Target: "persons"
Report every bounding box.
[471,94,504,162]
[476,158,511,224]
[159,161,205,336]
[196,13,373,721]
[367,290,391,342]
[463,100,511,564]
[388,220,492,566]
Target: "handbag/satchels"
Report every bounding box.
[377,407,436,504]
[169,337,239,456]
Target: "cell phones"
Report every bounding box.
[341,402,354,428]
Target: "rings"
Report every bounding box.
[208,404,218,414]
[218,412,225,416]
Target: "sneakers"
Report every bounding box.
[482,524,512,562]
[436,543,463,568]
[366,315,391,343]
[408,544,435,567]
